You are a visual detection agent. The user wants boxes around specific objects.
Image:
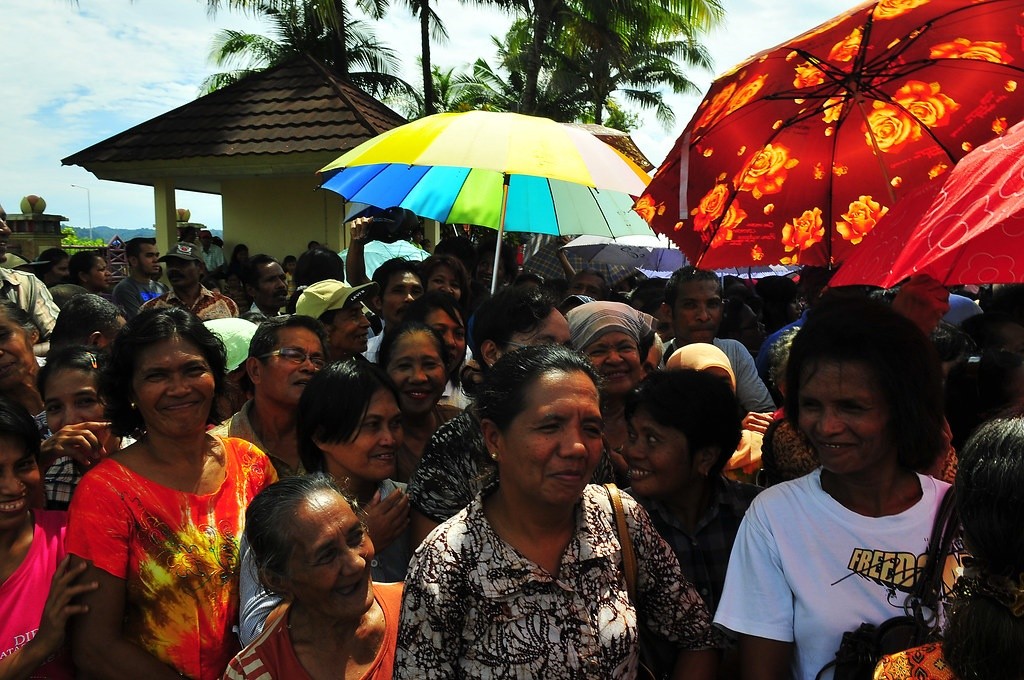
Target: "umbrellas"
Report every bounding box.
[314,110,663,294]
[630,0,1024,272]
[820,123,1024,296]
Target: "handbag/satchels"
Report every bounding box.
[605,483,677,680]
[816,487,961,680]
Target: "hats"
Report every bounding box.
[296,278,379,319]
[556,295,596,313]
[203,318,258,372]
[0,252,51,269]
[158,242,204,263]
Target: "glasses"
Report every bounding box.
[258,348,329,369]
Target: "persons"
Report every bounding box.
[64,308,279,680]
[224,475,403,680]
[0,206,1024,680]
[715,292,955,680]
[0,400,100,680]
[394,346,713,680]
[871,402,1024,680]
